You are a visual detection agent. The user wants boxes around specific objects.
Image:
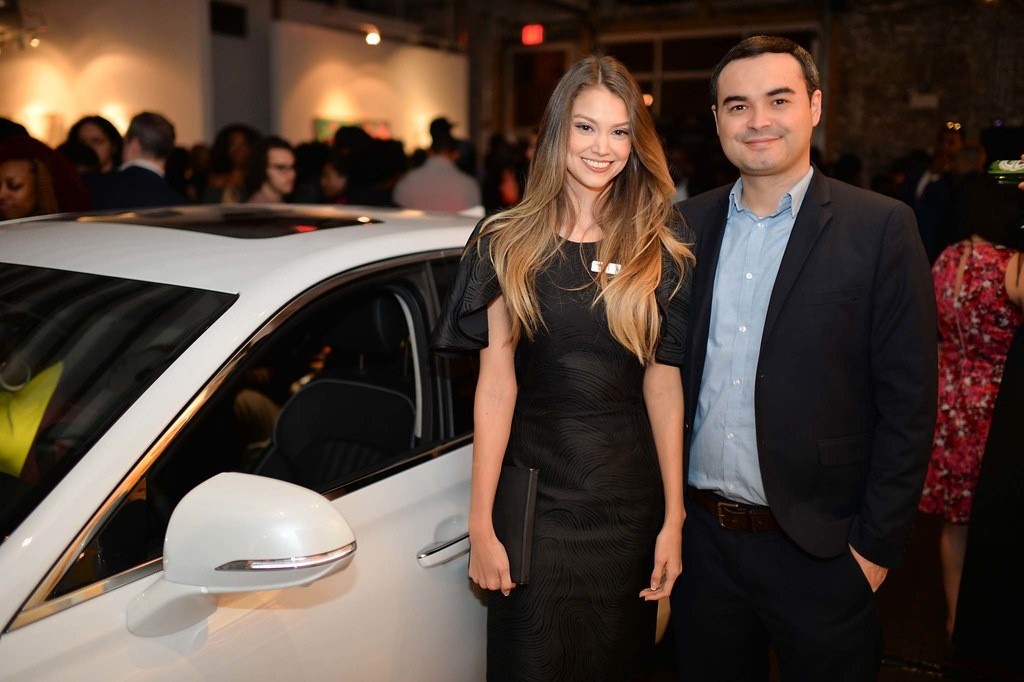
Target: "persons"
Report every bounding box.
[650,29,944,682]
[0,104,1024,222]
[914,182,1024,642]
[462,52,700,682]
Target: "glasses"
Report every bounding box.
[265,162,295,172]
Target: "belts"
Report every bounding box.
[690,489,778,532]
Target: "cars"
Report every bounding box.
[1,203,505,681]
